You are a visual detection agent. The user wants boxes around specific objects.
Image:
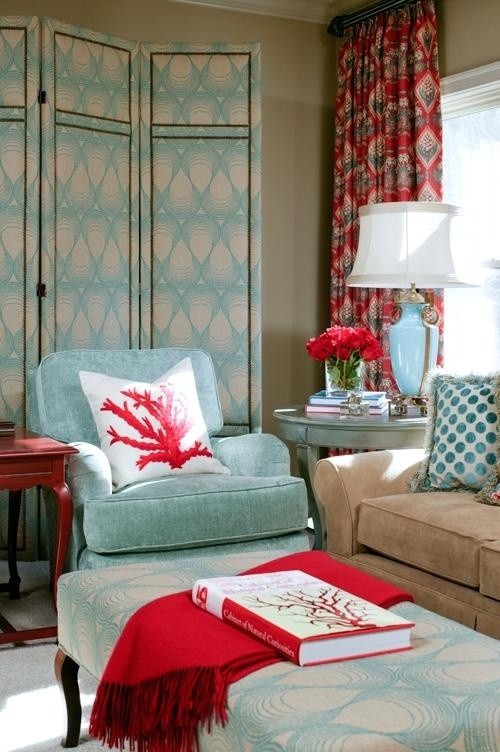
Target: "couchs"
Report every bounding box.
[313,448,498,640]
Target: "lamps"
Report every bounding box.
[346,200,478,418]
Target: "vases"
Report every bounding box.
[323,358,366,399]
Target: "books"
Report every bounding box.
[192,568,415,668]
[0,419,17,437]
[304,389,392,415]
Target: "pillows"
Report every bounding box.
[78,356,232,492]
[404,369,499,495]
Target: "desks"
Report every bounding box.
[271,405,428,551]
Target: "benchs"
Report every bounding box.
[52,551,500,752]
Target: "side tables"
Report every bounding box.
[0,425,80,649]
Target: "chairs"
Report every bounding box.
[29,341,312,568]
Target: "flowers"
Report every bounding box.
[306,324,384,391]
